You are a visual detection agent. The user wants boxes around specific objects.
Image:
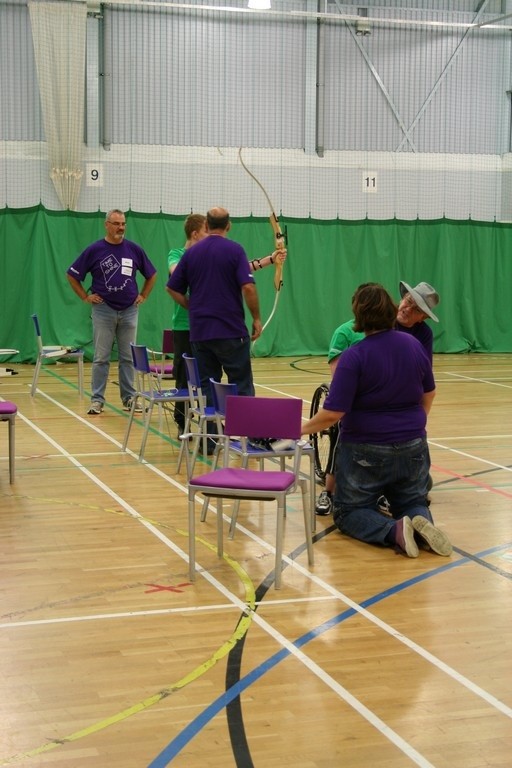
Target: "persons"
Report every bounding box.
[166,207,263,456]
[300,281,453,559]
[65,209,157,414]
[168,214,288,442]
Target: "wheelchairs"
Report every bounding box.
[309,383,342,487]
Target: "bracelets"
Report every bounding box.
[270,255,274,264]
[249,260,256,271]
[255,258,262,268]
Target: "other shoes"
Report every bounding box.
[199,444,213,455]
[178,426,193,441]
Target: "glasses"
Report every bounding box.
[107,221,127,227]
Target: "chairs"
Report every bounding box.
[0,400,18,484]
[29,312,317,591]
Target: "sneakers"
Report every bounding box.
[392,515,418,558]
[122,398,149,412]
[377,496,390,511]
[87,401,104,415]
[314,490,333,515]
[412,515,452,556]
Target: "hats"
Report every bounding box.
[399,282,440,323]
